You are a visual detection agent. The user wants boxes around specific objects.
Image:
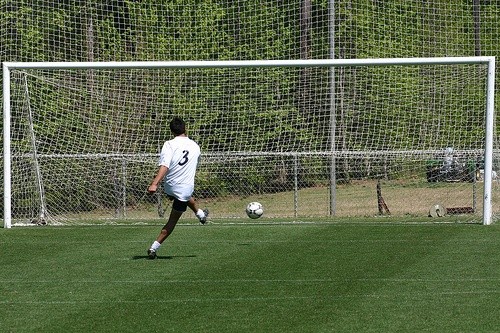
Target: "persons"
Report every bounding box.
[146,117,209,259]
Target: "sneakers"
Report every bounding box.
[147,248,157,260]
[199,208,209,225]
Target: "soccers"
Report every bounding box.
[246,202,264,219]
